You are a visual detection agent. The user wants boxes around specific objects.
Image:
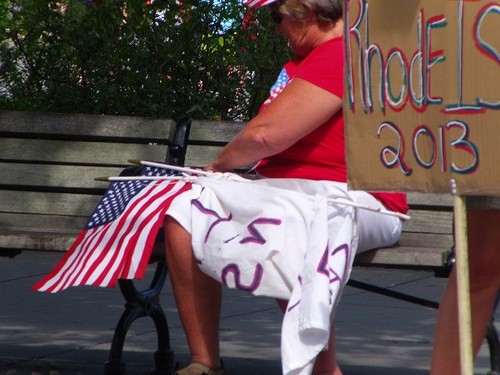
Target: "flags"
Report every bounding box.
[33,164,197,292]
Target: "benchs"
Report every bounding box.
[0,106,500,374]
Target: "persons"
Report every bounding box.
[431,195,500,375]
[160,0,408,375]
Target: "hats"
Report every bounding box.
[241,0,281,40]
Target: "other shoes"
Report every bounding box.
[176,356,224,375]
